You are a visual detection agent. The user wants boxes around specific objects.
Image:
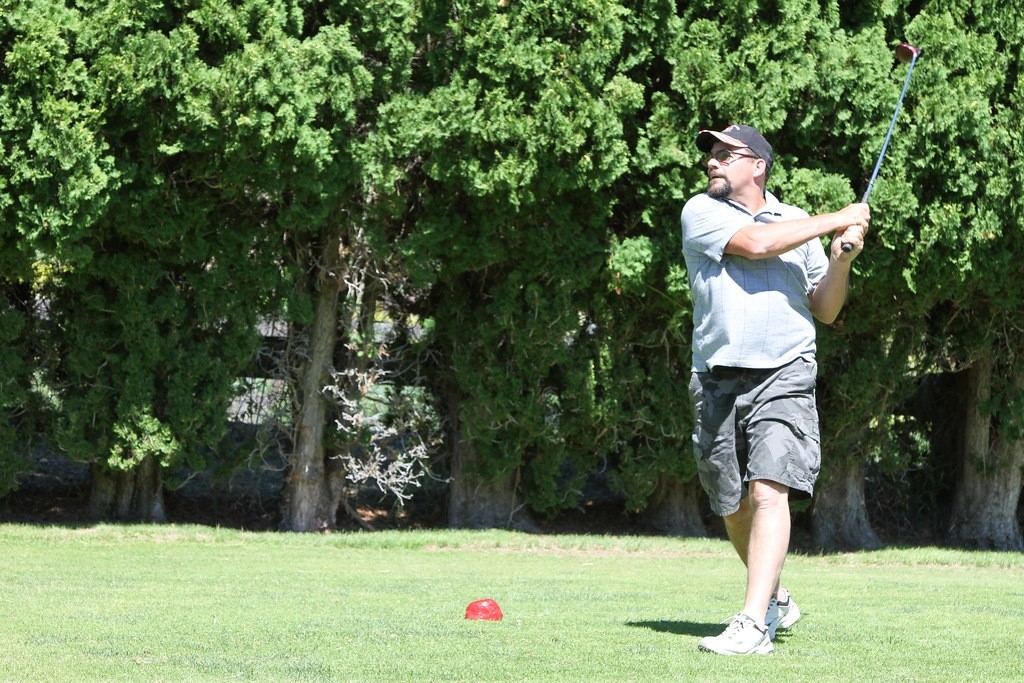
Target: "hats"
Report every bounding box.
[695,125,774,173]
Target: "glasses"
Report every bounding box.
[699,150,759,168]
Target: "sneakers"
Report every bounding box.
[698,610,774,657]
[762,588,801,641]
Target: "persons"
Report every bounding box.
[680,124,871,657]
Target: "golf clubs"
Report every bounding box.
[842,44,920,253]
